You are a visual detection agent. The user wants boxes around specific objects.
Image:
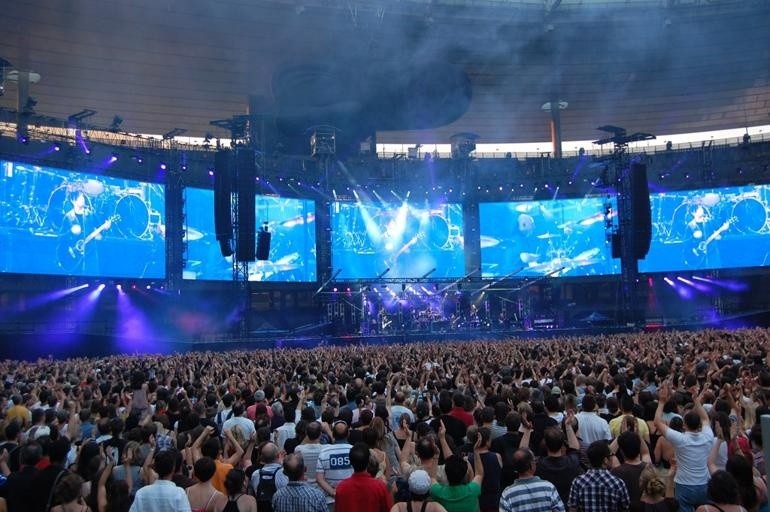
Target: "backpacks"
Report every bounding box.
[218,410,233,434]
[256,467,281,512]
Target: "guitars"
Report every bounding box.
[58,212,122,269]
[682,215,738,269]
[372,230,426,275]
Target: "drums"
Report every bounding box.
[419,213,452,250]
[723,196,767,235]
[101,193,151,238]
[571,248,610,275]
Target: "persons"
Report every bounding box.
[375,218,411,279]
[1,326,770,512]
[58,190,111,276]
[684,204,723,270]
[515,214,542,273]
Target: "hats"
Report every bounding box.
[551,386,561,395]
[254,390,265,401]
[408,470,432,494]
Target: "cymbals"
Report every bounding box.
[529,258,600,269]
[536,233,561,240]
[257,264,298,272]
[281,212,313,227]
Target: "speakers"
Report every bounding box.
[236,148,256,262]
[646,192,652,253]
[631,163,647,259]
[611,234,620,258]
[256,231,271,260]
[214,150,233,256]
